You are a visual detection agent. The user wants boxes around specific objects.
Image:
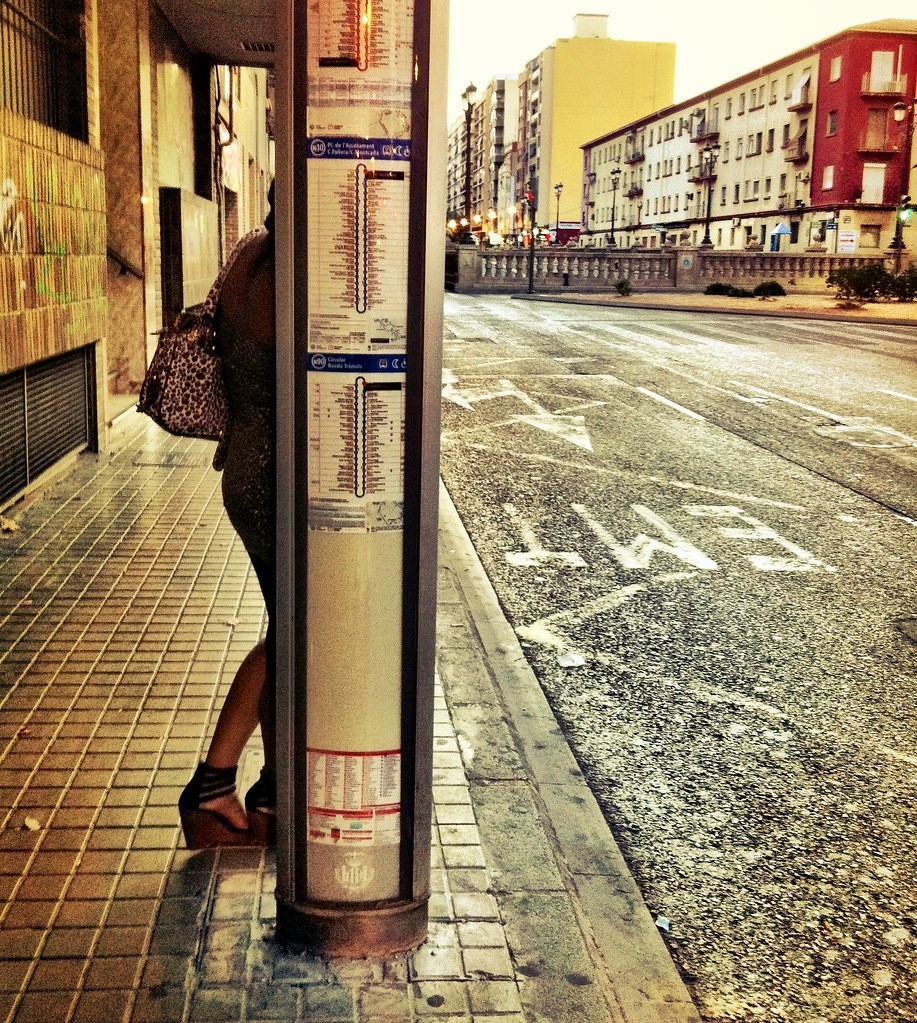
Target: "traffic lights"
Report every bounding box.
[526,177,540,213]
[898,195,914,228]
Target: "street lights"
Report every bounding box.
[555,182,563,244]
[701,144,721,243]
[461,82,477,245]
[606,167,621,247]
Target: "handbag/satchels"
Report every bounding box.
[136,225,270,439]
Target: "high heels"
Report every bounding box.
[245,765,277,846]
[178,761,261,850]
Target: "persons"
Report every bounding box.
[177,179,278,851]
